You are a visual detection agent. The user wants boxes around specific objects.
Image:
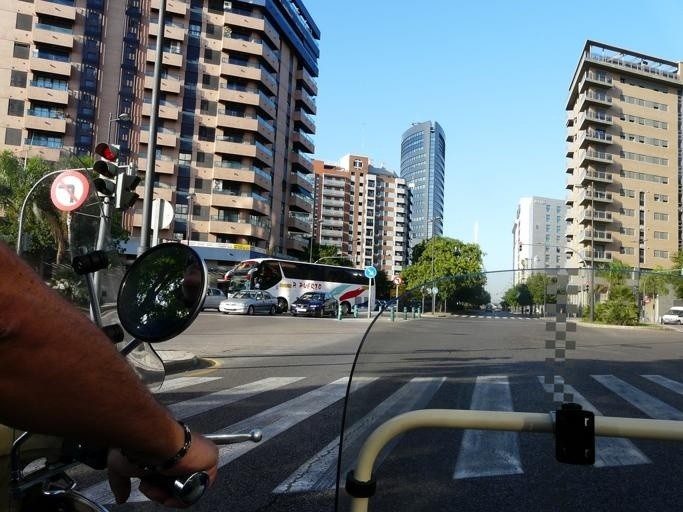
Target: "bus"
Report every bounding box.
[224,257,376,316]
[486,305,492,313]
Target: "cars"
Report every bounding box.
[201,288,279,316]
[290,292,338,318]
[495,308,501,312]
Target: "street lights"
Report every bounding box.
[430,217,442,314]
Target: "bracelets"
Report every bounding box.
[118,419,190,471]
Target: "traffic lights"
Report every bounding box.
[93,142,141,211]
[518,241,523,252]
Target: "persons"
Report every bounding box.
[0,244,220,508]
[181,263,201,302]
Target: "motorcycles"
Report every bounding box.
[1,241,263,512]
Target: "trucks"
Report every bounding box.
[662,306,683,325]
